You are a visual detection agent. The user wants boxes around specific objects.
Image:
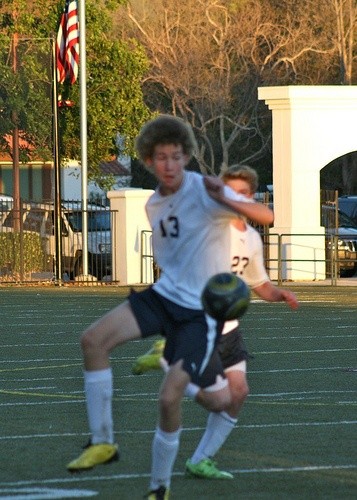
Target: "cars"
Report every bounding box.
[247,184,273,232]
[338,195,357,225]
[0,196,14,226]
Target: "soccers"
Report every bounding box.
[201,273,250,321]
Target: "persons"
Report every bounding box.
[131,164,297,479]
[66,114,274,500]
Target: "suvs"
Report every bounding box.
[320,205,357,277]
[0,199,112,282]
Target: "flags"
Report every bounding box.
[51,0,81,107]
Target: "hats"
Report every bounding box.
[134,115,194,172]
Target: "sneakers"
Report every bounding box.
[143,484,172,500]
[63,440,121,474]
[132,339,168,375]
[184,453,235,481]
